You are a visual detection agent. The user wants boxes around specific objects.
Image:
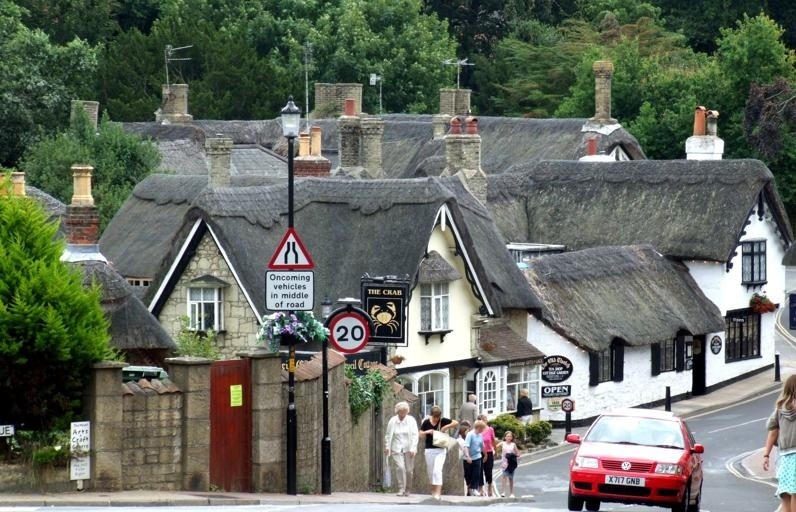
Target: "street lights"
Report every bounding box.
[317,294,333,495]
[277,90,304,496]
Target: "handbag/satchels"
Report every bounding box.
[431,418,457,450]
[382,447,391,490]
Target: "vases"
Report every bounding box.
[272,332,307,348]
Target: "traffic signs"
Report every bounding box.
[559,398,574,415]
[328,311,370,354]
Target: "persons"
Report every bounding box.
[762,374,796,512]
[516,389,532,441]
[383,394,519,499]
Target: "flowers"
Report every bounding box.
[253,305,333,360]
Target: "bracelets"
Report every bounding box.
[764,455,769,457]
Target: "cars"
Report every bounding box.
[564,406,709,512]
